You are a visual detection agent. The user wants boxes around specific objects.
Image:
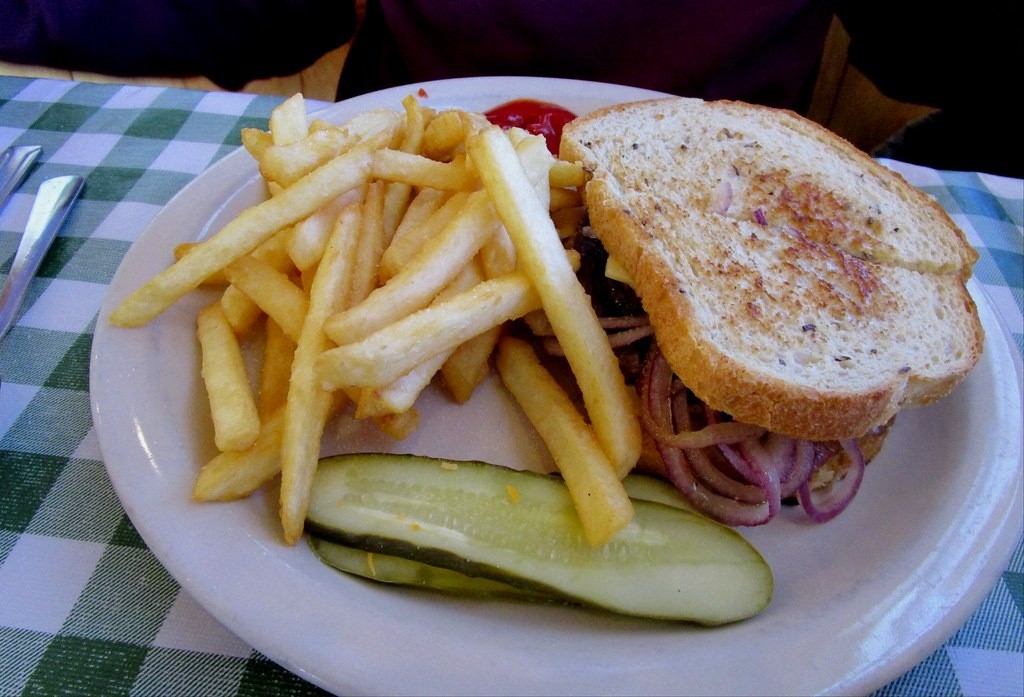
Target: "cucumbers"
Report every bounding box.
[279,447,777,626]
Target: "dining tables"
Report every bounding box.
[0,66,1024,697]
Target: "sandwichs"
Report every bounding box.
[559,96,988,526]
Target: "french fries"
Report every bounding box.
[103,86,636,550]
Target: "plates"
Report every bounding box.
[87,74,1022,697]
